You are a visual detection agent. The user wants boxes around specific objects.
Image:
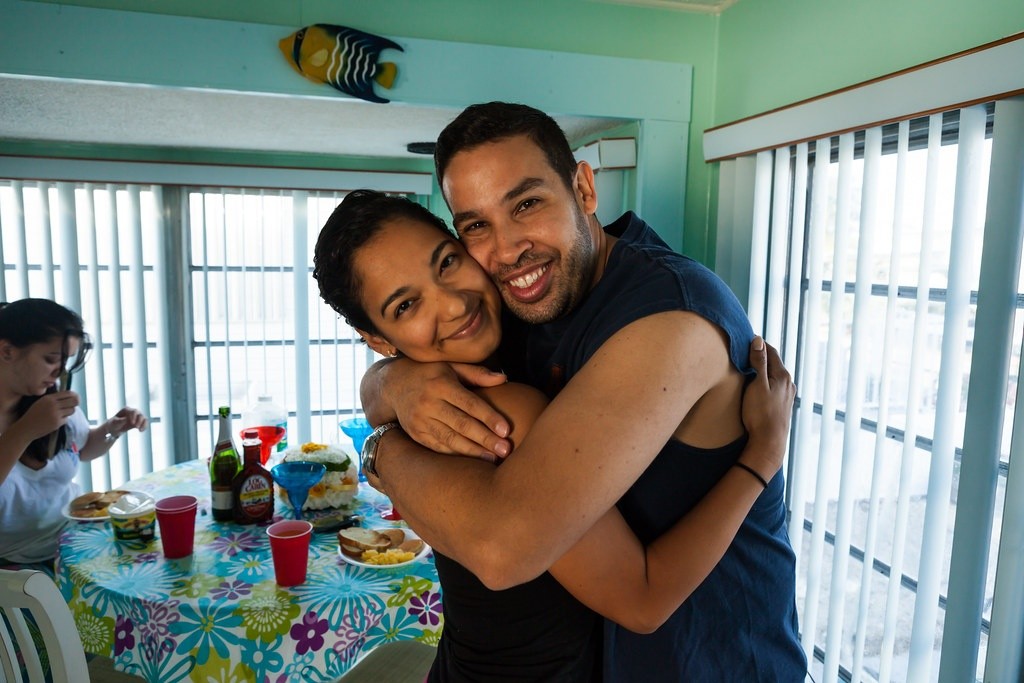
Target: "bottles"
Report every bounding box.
[243,394,288,472]
[230,430,274,526]
[209,407,242,522]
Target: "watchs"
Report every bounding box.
[361,422,398,478]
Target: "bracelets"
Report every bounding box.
[737,463,768,488]
[105,434,118,441]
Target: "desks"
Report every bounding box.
[54,445,445,683]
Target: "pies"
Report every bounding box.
[68,490,131,509]
[397,539,423,555]
[382,528,405,548]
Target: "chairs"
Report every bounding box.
[0,569,149,683]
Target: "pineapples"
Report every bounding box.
[360,547,416,565]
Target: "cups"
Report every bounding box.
[208,456,212,471]
[240,426,285,466]
[155,496,199,559]
[266,520,313,586]
[108,500,155,541]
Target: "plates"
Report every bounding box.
[61,490,154,520]
[338,527,432,568]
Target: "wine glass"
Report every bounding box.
[271,462,327,541]
[340,418,374,482]
[380,505,402,520]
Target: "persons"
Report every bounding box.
[309,186,794,683]
[361,95,808,683]
[0,298,148,668]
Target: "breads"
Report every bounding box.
[338,527,391,557]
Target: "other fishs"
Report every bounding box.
[277,23,405,106]
